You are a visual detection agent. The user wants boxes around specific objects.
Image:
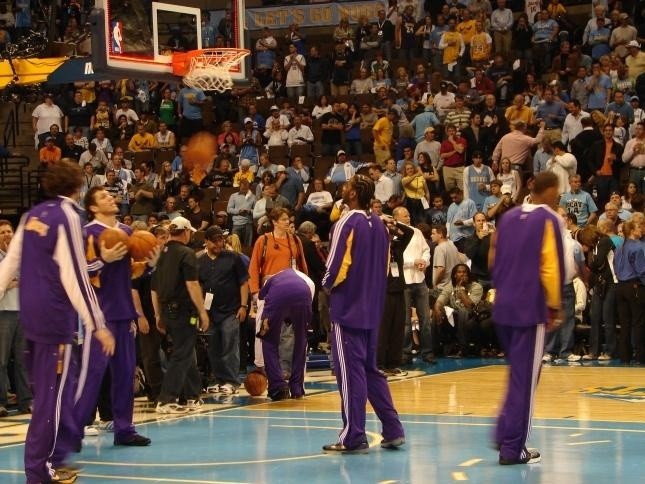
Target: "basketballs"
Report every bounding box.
[98,228,130,249]
[245,373,267,396]
[182,131,217,170]
[129,230,157,259]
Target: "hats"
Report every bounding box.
[337,149,346,157]
[625,40,642,48]
[244,117,253,124]
[171,217,198,233]
[275,165,288,173]
[216,210,228,217]
[619,12,628,19]
[206,224,225,240]
[424,126,435,134]
[270,105,279,112]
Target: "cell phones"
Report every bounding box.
[483,223,489,231]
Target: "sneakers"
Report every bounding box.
[106,421,115,430]
[269,386,289,401]
[207,384,221,393]
[52,471,76,484]
[188,398,204,410]
[411,344,420,354]
[384,367,408,377]
[18,407,31,413]
[500,448,541,464]
[423,344,505,363]
[83,425,99,435]
[401,358,412,364]
[381,436,406,447]
[156,400,189,414]
[323,441,368,454]
[124,435,151,445]
[0,406,7,415]
[220,383,239,395]
[542,350,613,361]
[624,359,630,366]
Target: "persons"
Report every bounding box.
[0,0,644,415]
[488,167,580,466]
[321,172,409,454]
[72,183,162,447]
[0,155,119,482]
